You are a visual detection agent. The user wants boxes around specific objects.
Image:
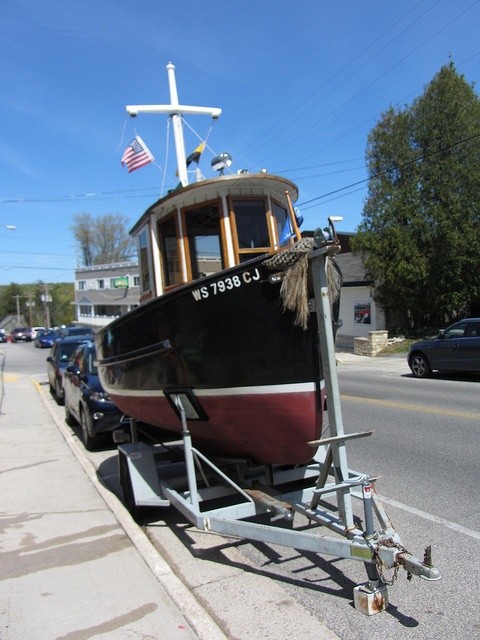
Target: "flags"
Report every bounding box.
[120,139,151,173]
[174,141,205,175]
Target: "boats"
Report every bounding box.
[97,61,441,587]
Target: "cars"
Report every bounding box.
[12,328,32,343]
[32,327,45,339]
[47,334,96,405]
[62,340,139,449]
[408,318,480,377]
[34,330,60,347]
[64,327,95,337]
